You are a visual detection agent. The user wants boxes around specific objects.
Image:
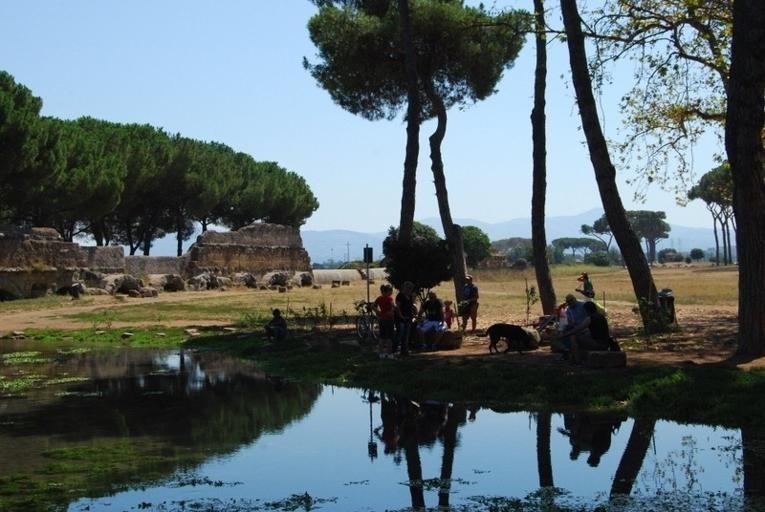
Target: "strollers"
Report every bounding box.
[534,303,567,344]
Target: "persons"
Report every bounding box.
[263,309,287,343]
[567,301,610,369]
[460,275,480,331]
[370,282,455,357]
[561,294,590,346]
[578,272,594,302]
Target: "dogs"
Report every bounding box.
[476,323,537,354]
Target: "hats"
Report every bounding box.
[465,275,472,280]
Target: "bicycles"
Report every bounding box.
[356,301,379,341]
